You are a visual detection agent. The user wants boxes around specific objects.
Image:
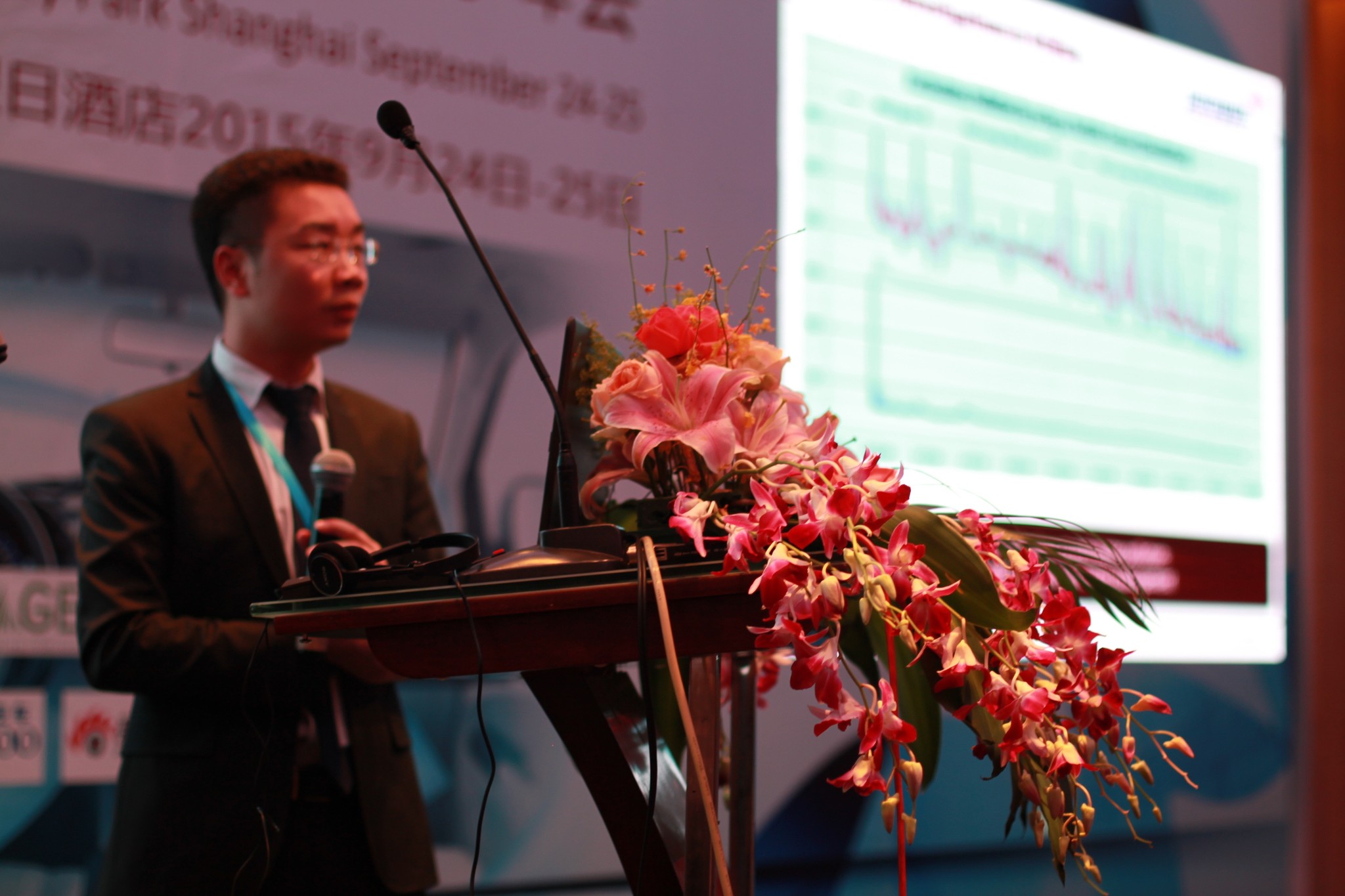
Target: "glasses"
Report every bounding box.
[235,233,380,266]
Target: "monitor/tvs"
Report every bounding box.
[533,310,637,556]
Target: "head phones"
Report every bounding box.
[308,529,483,597]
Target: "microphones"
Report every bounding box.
[377,99,628,559]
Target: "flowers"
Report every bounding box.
[579,178,1200,896]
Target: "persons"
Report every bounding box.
[76,147,448,896]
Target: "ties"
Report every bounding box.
[263,382,340,782]
